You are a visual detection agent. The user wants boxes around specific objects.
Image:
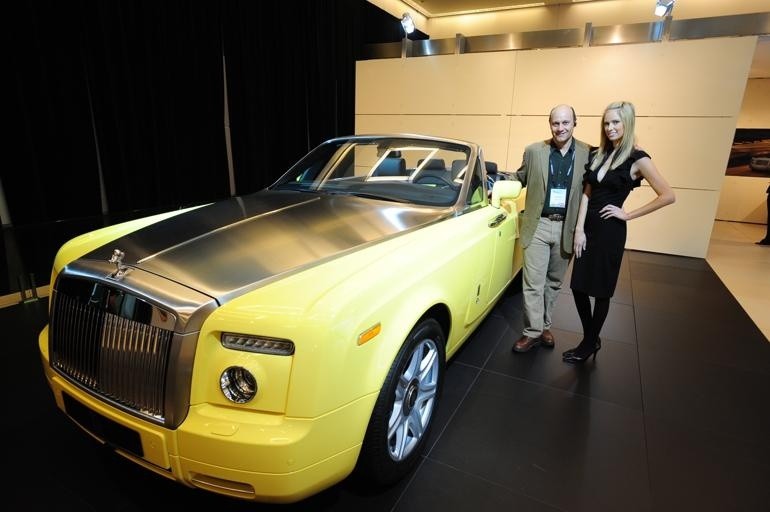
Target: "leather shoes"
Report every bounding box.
[512,336,541,352]
[541,331,554,347]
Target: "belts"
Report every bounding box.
[540,213,564,222]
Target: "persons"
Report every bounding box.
[754,184,769,245]
[486,103,644,355]
[562,100,677,365]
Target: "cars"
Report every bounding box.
[750,151,770,171]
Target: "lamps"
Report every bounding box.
[400,13,415,34]
[654,0,675,17]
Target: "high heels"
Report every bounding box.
[563,337,601,364]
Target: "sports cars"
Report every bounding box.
[37,132,526,504]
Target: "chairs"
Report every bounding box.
[452,160,497,185]
[417,159,445,182]
[372,151,406,176]
